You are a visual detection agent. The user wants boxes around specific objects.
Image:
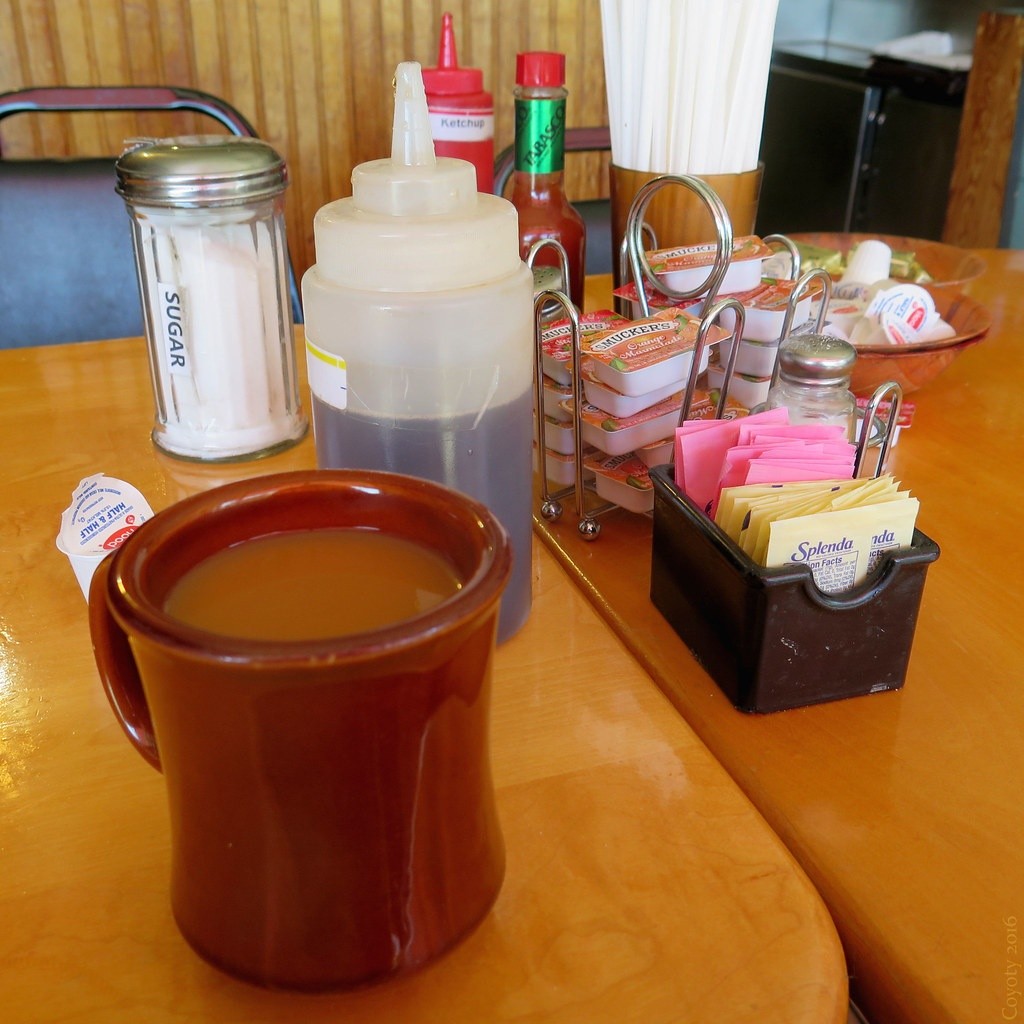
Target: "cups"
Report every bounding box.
[90,469,511,994]
[610,160,764,316]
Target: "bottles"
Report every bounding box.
[767,334,857,443]
[419,12,493,197]
[531,265,580,326]
[510,52,586,318]
[304,62,533,648]
[114,136,308,463]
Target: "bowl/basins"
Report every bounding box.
[815,274,993,398]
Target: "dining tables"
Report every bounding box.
[536,232,1018,1017]
[0,327,848,1022]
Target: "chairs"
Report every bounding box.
[493,128,615,277]
[1,83,304,357]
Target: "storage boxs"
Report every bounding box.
[650,458,939,715]
